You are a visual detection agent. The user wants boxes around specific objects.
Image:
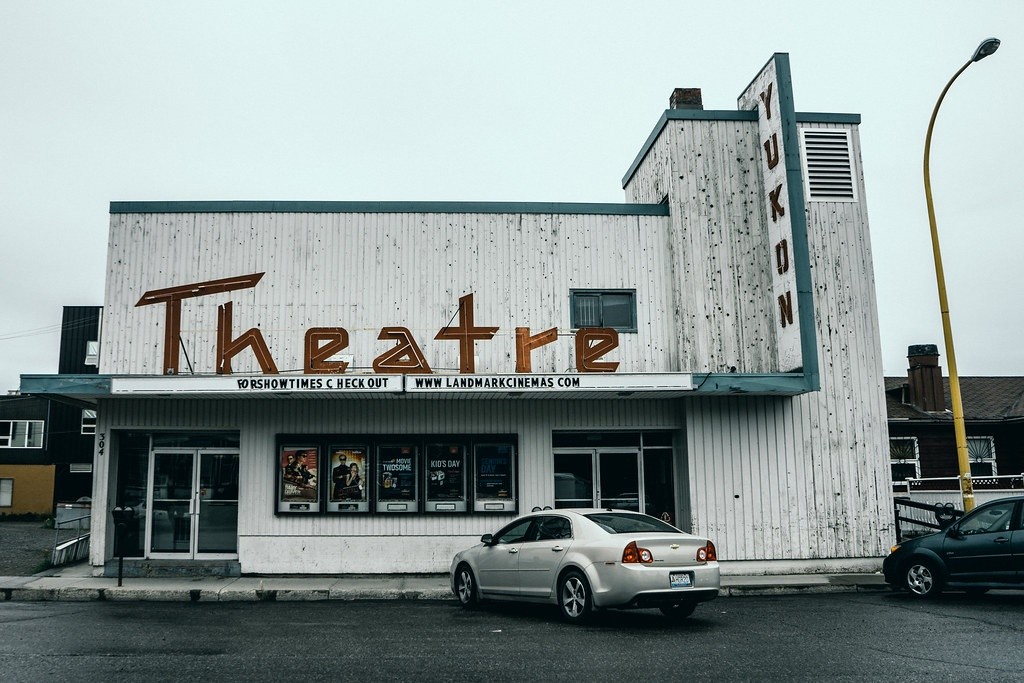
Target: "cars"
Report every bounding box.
[449,508,721,622]
[883,496,1024,601]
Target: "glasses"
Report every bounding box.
[287,458,293,460]
[300,455,308,458]
[340,458,346,461]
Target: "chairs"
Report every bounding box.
[537,521,561,540]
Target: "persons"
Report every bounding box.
[284,451,316,487]
[332,454,364,500]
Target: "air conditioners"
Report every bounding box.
[892,481,911,499]
